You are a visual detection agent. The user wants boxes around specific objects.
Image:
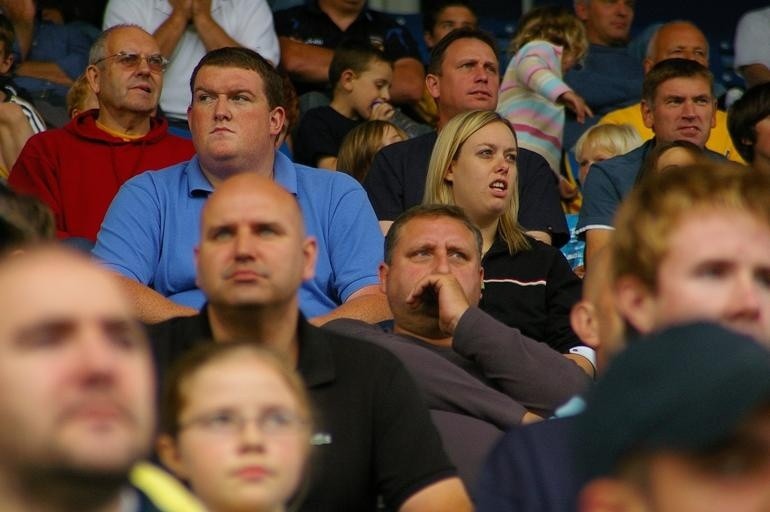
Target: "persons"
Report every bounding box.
[0,1,770,512]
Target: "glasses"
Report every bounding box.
[173,407,313,447]
[94,50,169,75]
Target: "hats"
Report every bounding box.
[599,321,766,469]
